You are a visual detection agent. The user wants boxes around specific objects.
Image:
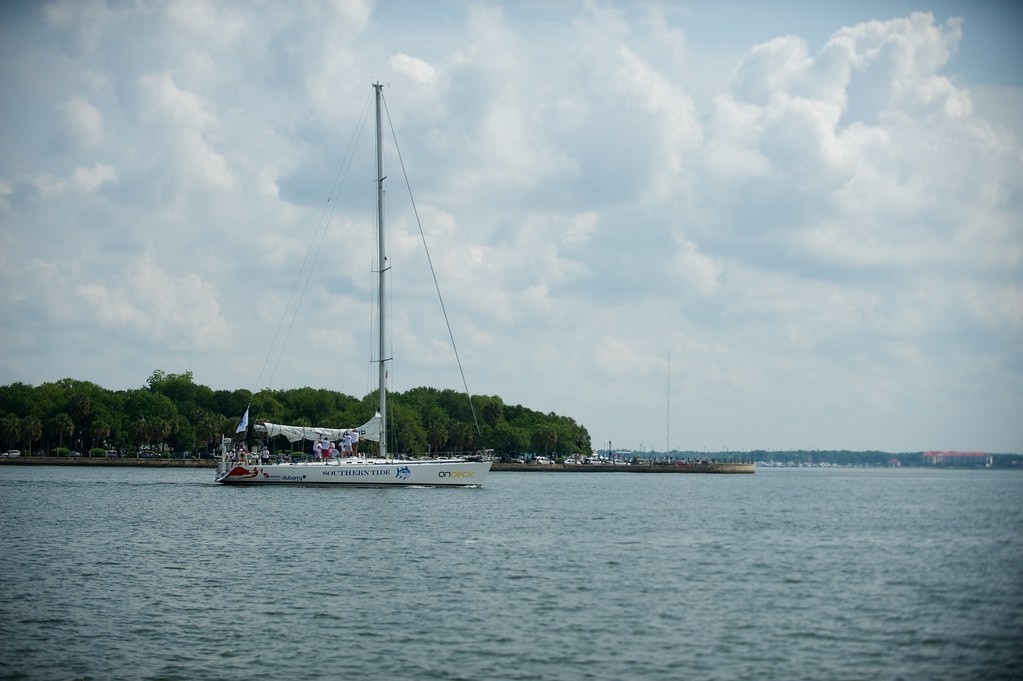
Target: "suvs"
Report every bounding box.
[531,456,556,465]
[107,450,117,459]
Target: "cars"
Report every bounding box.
[139,451,162,458]
[564,458,581,466]
[2,449,20,456]
[70,450,80,457]
[582,454,631,466]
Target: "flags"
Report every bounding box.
[236,409,249,433]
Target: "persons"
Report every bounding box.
[229,444,270,465]
[313,430,359,462]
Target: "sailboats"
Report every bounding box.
[213,79,496,486]
